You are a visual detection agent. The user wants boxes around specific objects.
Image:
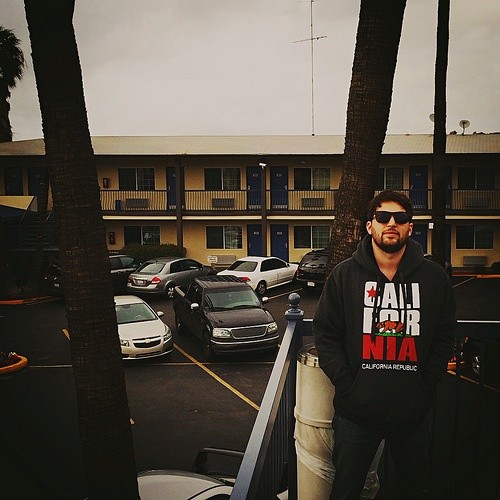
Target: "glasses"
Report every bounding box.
[373,211,412,224]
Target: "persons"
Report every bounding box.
[312,190,458,500]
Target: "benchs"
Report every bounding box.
[301,198,325,210]
[210,255,236,272]
[464,198,490,209]
[125,199,149,210]
[212,199,235,210]
[463,256,488,267]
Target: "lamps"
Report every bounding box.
[108,232,115,244]
[103,178,109,188]
